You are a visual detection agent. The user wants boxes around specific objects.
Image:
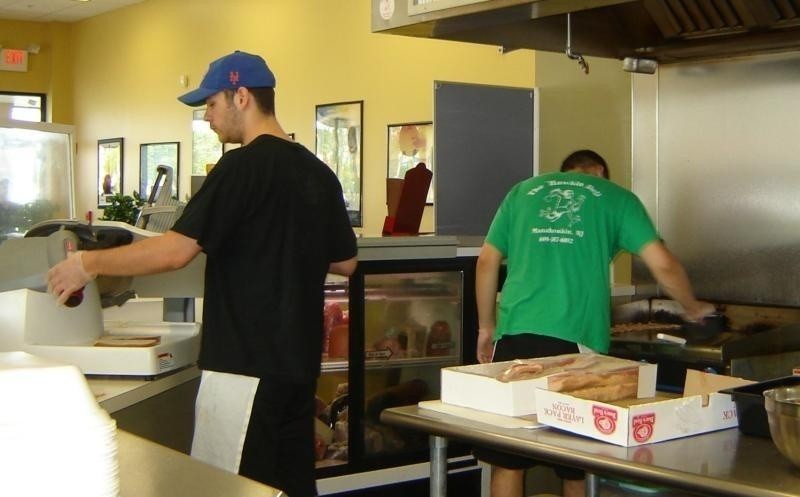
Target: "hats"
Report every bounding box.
[177,49,276,108]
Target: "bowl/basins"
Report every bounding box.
[761,385,800,471]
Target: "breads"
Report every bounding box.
[497,354,639,403]
[94,335,160,347]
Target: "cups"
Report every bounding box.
[398,323,427,358]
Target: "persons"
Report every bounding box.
[474,149,718,497]
[39,48,361,496]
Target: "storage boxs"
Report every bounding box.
[438,347,761,451]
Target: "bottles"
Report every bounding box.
[57,238,84,307]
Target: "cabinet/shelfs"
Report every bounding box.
[314,235,511,497]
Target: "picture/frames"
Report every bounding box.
[386,120,435,206]
[191,108,224,177]
[314,100,365,228]
[97,137,124,210]
[139,142,180,205]
